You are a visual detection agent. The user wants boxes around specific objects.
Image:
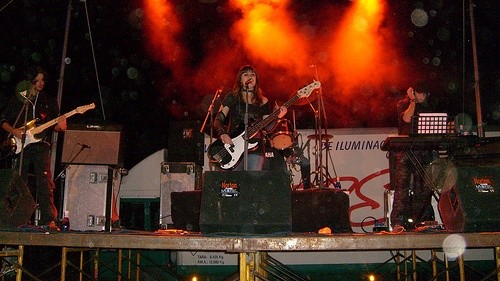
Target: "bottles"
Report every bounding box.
[336,181,341,189]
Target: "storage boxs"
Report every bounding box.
[63,165,113,232]
[159,162,199,229]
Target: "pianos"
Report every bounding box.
[381,135,480,151]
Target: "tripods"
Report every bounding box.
[293,87,339,191]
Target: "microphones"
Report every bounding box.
[32,80,37,83]
[199,169,292,236]
[244,79,252,85]
[78,143,90,148]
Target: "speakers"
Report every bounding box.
[0,169,37,229]
[439,165,500,233]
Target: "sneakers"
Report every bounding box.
[392,224,404,232]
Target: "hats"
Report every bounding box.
[411,78,429,93]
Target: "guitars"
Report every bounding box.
[11,102,95,154]
[207,81,321,170]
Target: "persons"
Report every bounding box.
[0,68,68,231]
[264,98,311,189]
[213,64,288,171]
[390,82,433,231]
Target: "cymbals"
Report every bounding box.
[290,89,317,105]
[307,134,333,140]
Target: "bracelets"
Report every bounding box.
[410,98,417,102]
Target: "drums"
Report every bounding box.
[269,119,294,150]
